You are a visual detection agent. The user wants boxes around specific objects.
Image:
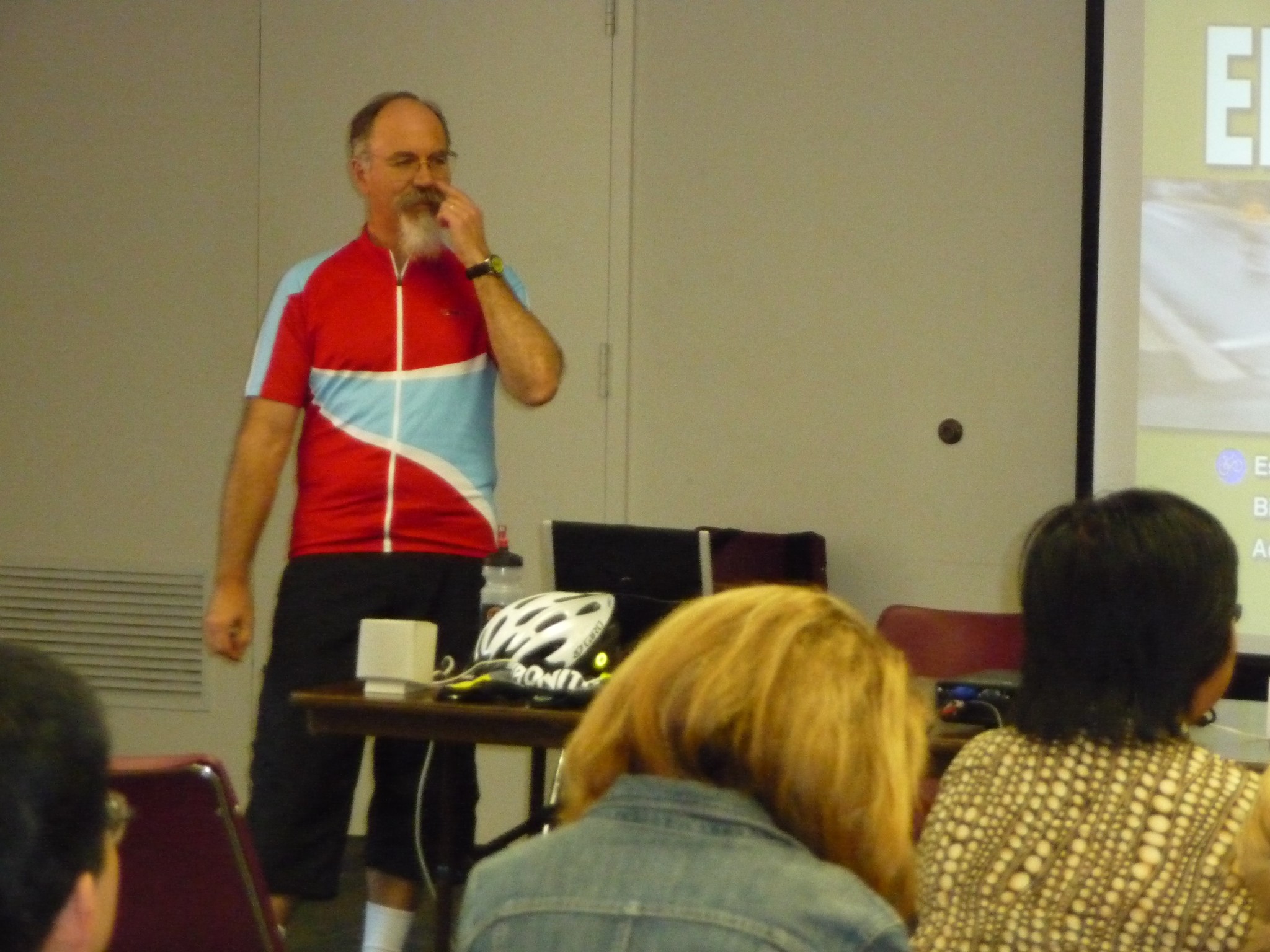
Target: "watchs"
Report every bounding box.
[465,253,505,282]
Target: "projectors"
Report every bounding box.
[935,667,1024,725]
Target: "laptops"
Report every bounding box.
[541,519,711,681]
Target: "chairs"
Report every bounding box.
[106,752,285,950]
[541,520,1027,834]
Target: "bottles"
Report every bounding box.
[480,544,523,629]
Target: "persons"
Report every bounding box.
[204,89,563,951]
[906,488,1270,952]
[446,584,935,952]
[0,640,134,952]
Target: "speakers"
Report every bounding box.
[356,618,438,694]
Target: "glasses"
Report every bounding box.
[102,791,132,843]
[358,150,458,176]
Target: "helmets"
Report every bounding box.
[474,590,622,680]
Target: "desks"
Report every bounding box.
[290,654,1012,952]
